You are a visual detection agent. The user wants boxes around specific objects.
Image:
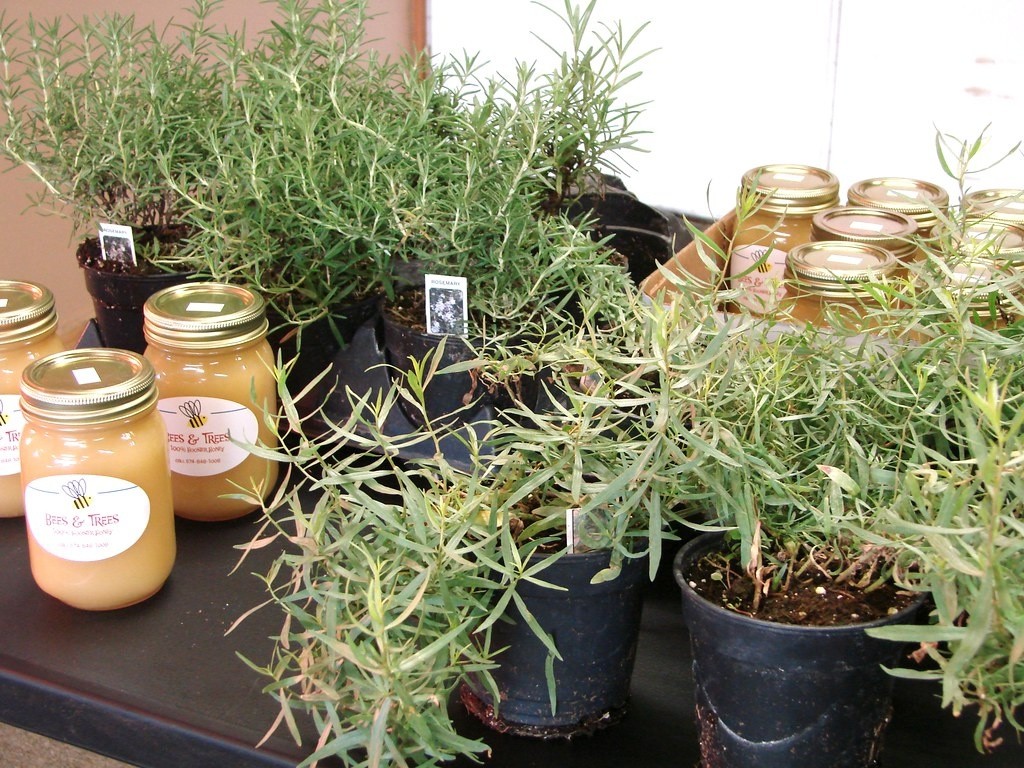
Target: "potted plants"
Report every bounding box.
[0,1,677,490]
[218,120,1023,768]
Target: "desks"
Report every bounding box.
[0,482,1024,768]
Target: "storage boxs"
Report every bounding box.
[642,210,920,360]
